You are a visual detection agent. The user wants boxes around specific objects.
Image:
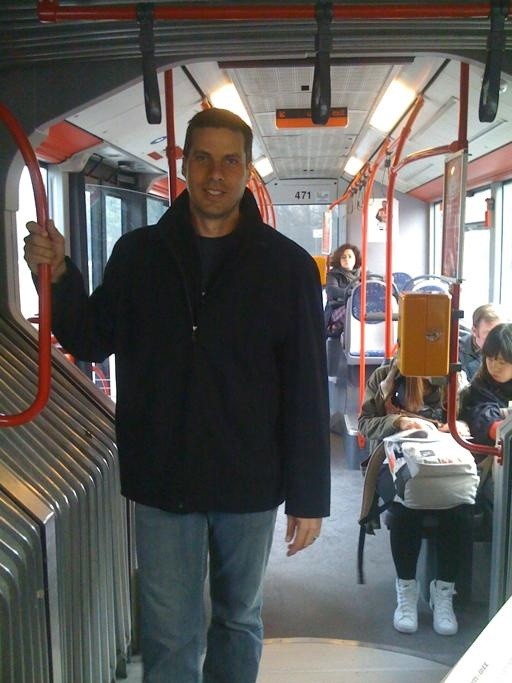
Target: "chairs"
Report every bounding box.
[341,271,455,366]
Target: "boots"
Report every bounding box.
[393,577,420,634]
[429,578,459,636]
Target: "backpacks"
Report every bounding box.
[358,419,480,532]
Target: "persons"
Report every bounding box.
[459,322,512,539]
[22,108,332,683]
[458,303,502,382]
[375,200,387,231]
[325,243,362,395]
[358,358,480,636]
